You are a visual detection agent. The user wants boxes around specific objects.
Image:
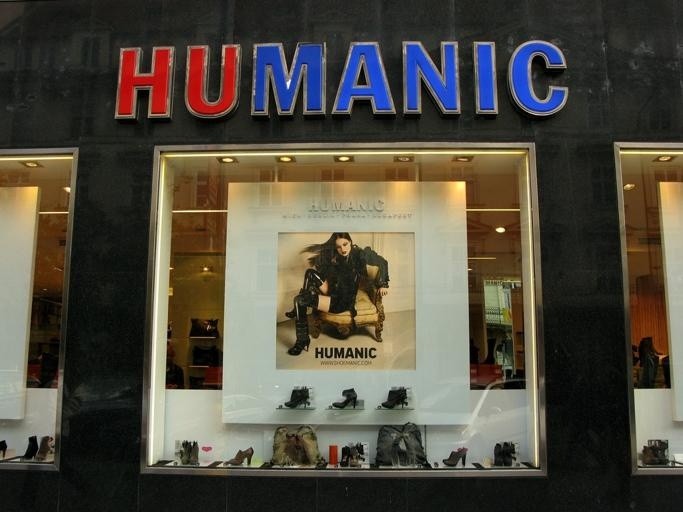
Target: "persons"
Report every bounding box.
[285,232,389,356]
[166,345,184,389]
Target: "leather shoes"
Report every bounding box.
[182,441,199,465]
[494,443,512,466]
[350,448,360,466]
[644,444,670,466]
[340,446,350,466]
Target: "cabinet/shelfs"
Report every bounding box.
[188,336,216,368]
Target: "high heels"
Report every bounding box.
[23,436,38,459]
[333,388,357,409]
[36,437,50,460]
[443,447,468,466]
[228,447,253,465]
[0,441,7,458]
[382,389,406,409]
[284,390,309,410]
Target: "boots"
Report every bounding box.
[289,321,311,356]
[284,290,307,318]
[483,339,497,364]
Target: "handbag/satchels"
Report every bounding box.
[189,317,219,338]
[190,346,223,366]
